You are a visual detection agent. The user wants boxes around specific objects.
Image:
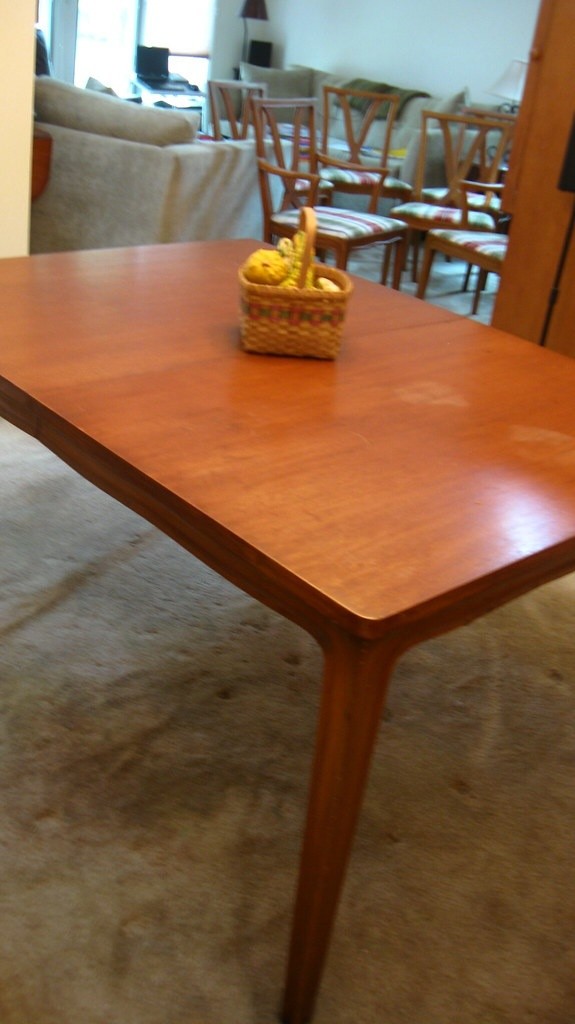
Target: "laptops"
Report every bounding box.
[136,45,184,91]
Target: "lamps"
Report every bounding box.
[236,0,269,62]
[490,58,528,114]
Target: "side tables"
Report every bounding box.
[459,130,511,172]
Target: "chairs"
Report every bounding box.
[208,78,517,314]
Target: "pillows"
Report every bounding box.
[428,89,469,129]
[240,62,313,124]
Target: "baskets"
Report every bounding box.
[239,203,357,357]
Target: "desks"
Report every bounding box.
[129,73,202,112]
[1,239,575,1023]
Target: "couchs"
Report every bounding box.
[28,74,294,255]
[202,63,519,218]
